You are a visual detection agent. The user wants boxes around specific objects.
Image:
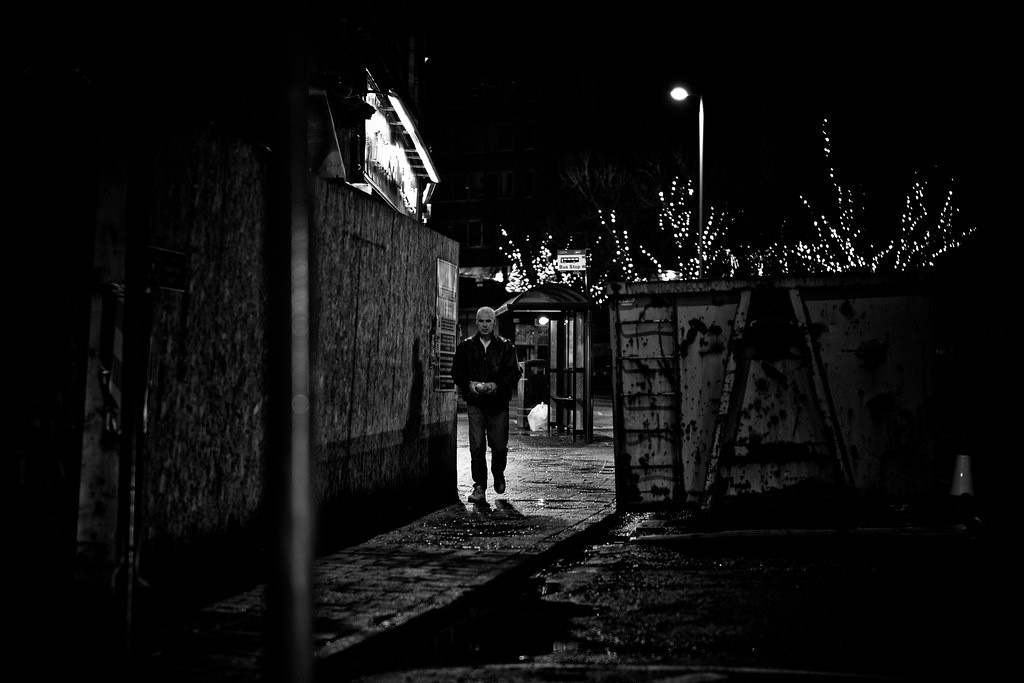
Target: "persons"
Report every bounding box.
[451,306,522,501]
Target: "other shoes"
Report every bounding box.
[468,483,486,503]
[493,474,505,494]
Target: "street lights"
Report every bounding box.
[670,87,704,279]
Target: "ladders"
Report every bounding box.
[606,280,686,513]
[703,278,861,518]
